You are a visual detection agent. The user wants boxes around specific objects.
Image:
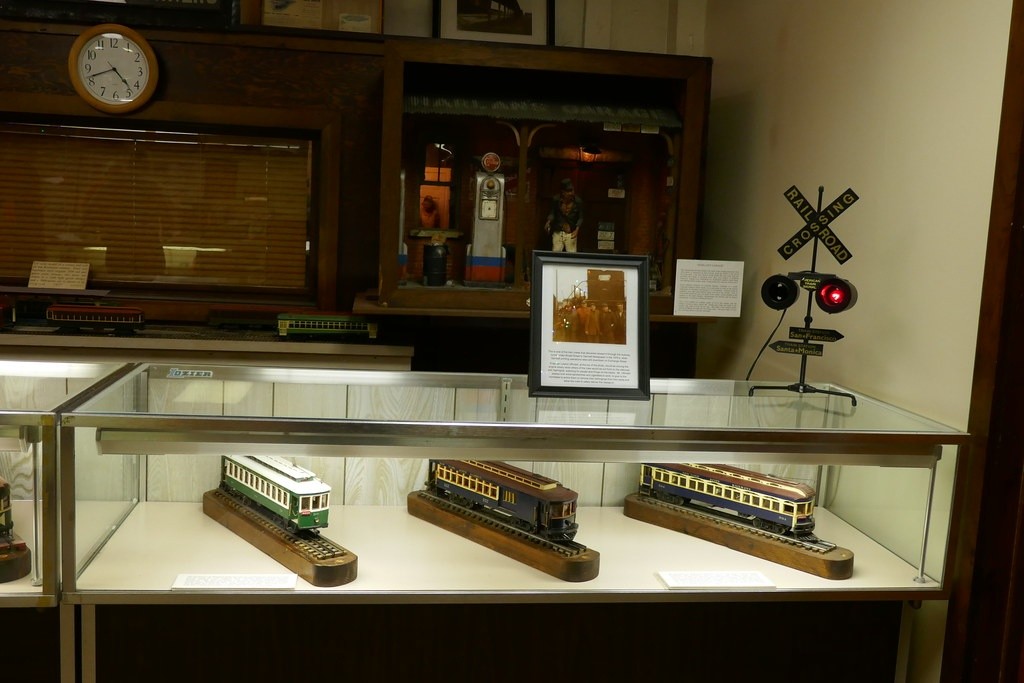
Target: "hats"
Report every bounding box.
[581,301,586,304]
[601,303,608,307]
[590,303,596,306]
[617,302,623,306]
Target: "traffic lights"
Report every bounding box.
[760,274,800,310]
[815,275,852,316]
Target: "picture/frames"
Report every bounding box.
[527,250,650,399]
[432,0,556,46]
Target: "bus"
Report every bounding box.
[638,464,816,536]
[425,459,579,541]
[272,313,382,345]
[45,302,147,336]
[219,453,332,534]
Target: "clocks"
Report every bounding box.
[68,23,159,113]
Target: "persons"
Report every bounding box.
[544,176,586,253]
[558,301,627,344]
[417,196,441,230]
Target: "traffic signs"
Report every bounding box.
[789,326,846,343]
[768,341,823,356]
